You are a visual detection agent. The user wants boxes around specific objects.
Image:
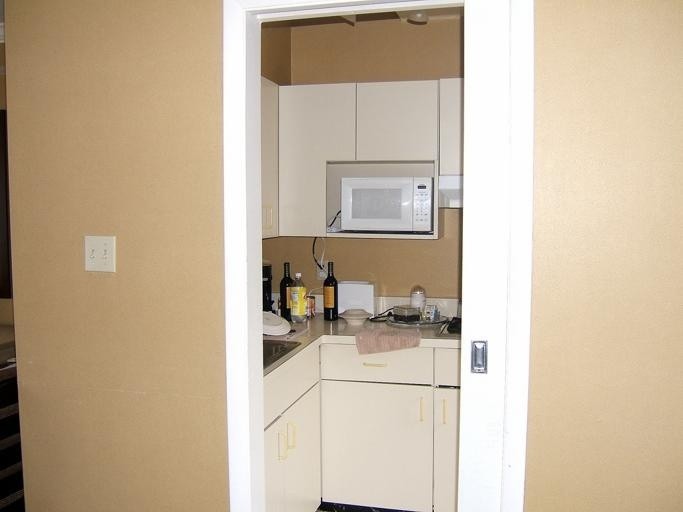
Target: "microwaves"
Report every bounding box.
[339,176,432,234]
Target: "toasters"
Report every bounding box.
[335,280,375,318]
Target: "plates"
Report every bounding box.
[337,313,374,326]
[262,311,291,336]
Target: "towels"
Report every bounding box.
[356,324,421,354]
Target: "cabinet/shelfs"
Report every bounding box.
[439,77,463,210]
[261,76,279,239]
[320,343,461,512]
[263,345,321,511]
[279,79,439,240]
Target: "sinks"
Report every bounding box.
[264,340,301,369]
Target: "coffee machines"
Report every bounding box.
[262,263,272,312]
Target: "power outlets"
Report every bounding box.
[316,260,329,280]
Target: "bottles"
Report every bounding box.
[322,261,338,320]
[290,271,307,324]
[279,261,293,322]
[409,285,427,315]
